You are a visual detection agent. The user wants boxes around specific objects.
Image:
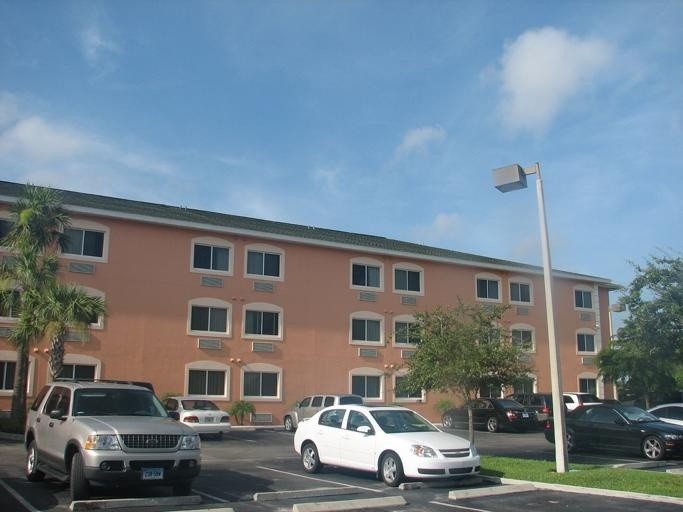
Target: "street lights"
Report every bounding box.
[492,160,570,473]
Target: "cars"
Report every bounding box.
[161,396,232,441]
[294,405,482,488]
[646,403,683,426]
[441,397,538,433]
[545,405,683,461]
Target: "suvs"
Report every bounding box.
[504,393,568,431]
[563,391,599,413]
[25,381,203,501]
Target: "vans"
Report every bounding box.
[283,394,364,433]
